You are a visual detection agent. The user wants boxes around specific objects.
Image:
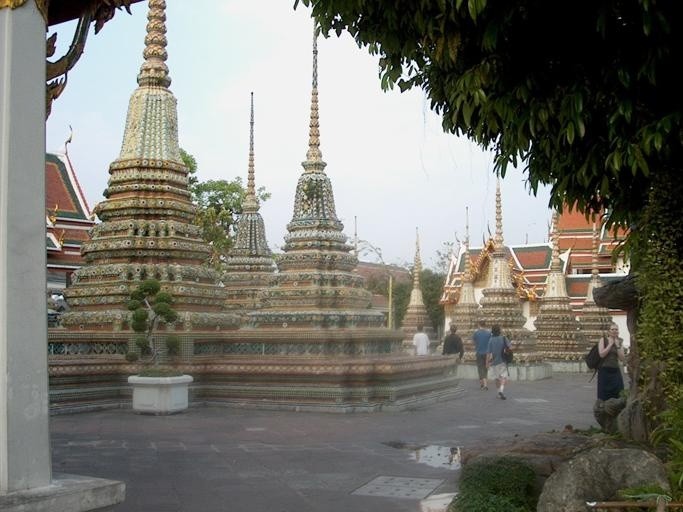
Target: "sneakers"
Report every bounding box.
[480,384,489,392]
[496,391,506,401]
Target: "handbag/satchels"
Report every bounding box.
[501,336,514,364]
[585,335,608,369]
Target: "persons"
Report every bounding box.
[596,322,625,401]
[472,319,491,390]
[484,323,512,400]
[412,323,431,356]
[440,323,465,361]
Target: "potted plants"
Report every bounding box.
[121,276,194,414]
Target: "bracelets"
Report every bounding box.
[615,346,621,349]
